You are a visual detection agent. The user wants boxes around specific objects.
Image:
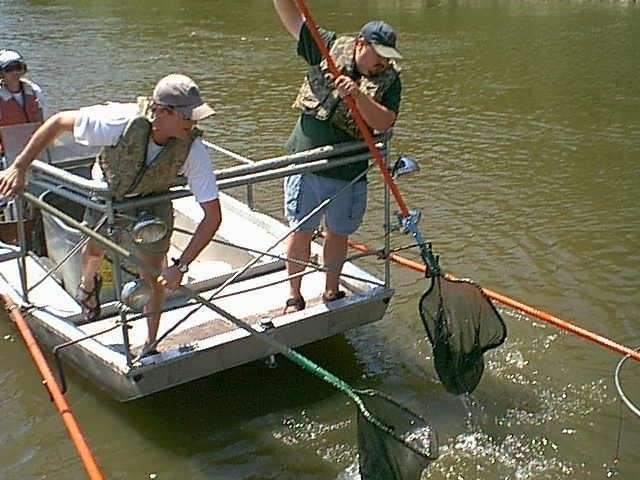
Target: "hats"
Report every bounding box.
[151,72,218,122]
[358,20,406,60]
[0,48,25,68]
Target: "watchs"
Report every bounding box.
[172,256,190,273]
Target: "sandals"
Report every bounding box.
[321,288,346,303]
[75,273,103,323]
[282,292,306,317]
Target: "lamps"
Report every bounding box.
[387,154,419,179]
[117,276,151,311]
[129,206,167,245]
[385,209,420,234]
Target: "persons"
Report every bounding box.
[273,1,406,316]
[0,47,53,167]
[1,72,222,357]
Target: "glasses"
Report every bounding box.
[4,63,22,73]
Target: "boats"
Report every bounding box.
[0,115,408,407]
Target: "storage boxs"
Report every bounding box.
[38,185,169,306]
